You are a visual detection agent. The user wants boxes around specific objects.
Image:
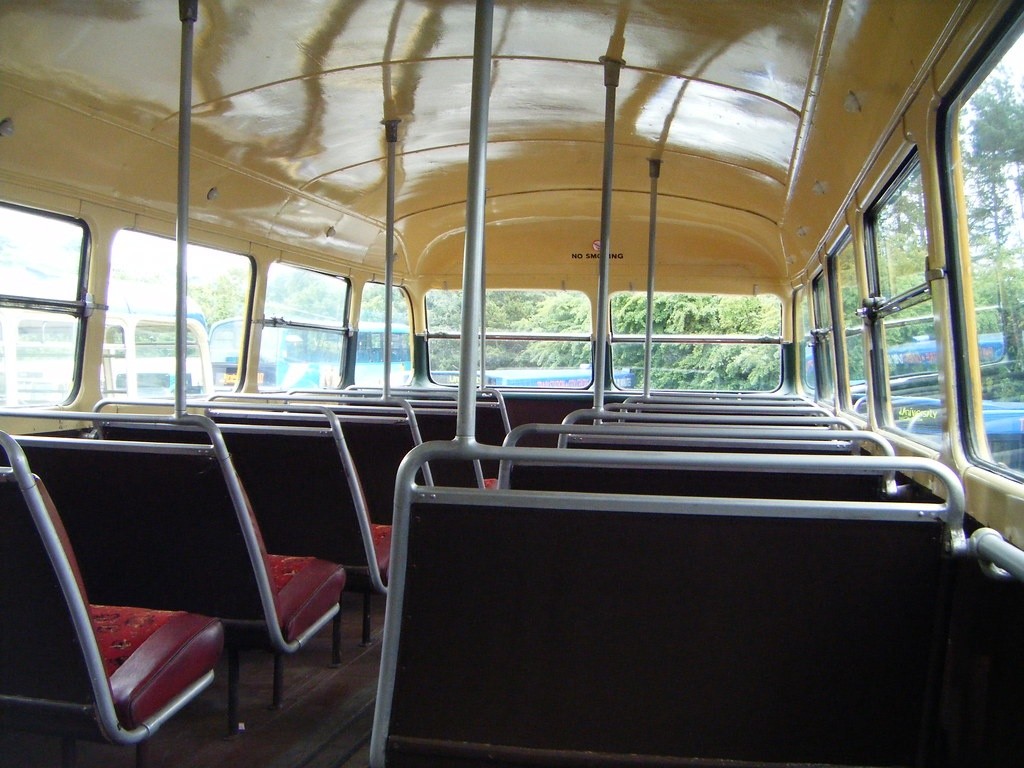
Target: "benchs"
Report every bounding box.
[0,388,967,766]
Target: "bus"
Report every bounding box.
[861,398,1024,472]
[209,316,412,391]
[0,314,215,405]
[887,332,1005,374]
[405,365,634,387]
